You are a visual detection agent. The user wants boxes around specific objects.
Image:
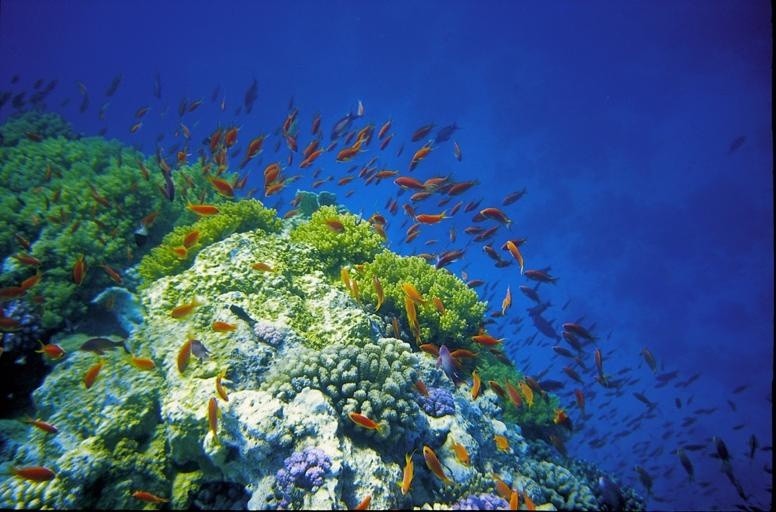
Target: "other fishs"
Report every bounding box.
[0,70,773,511]
